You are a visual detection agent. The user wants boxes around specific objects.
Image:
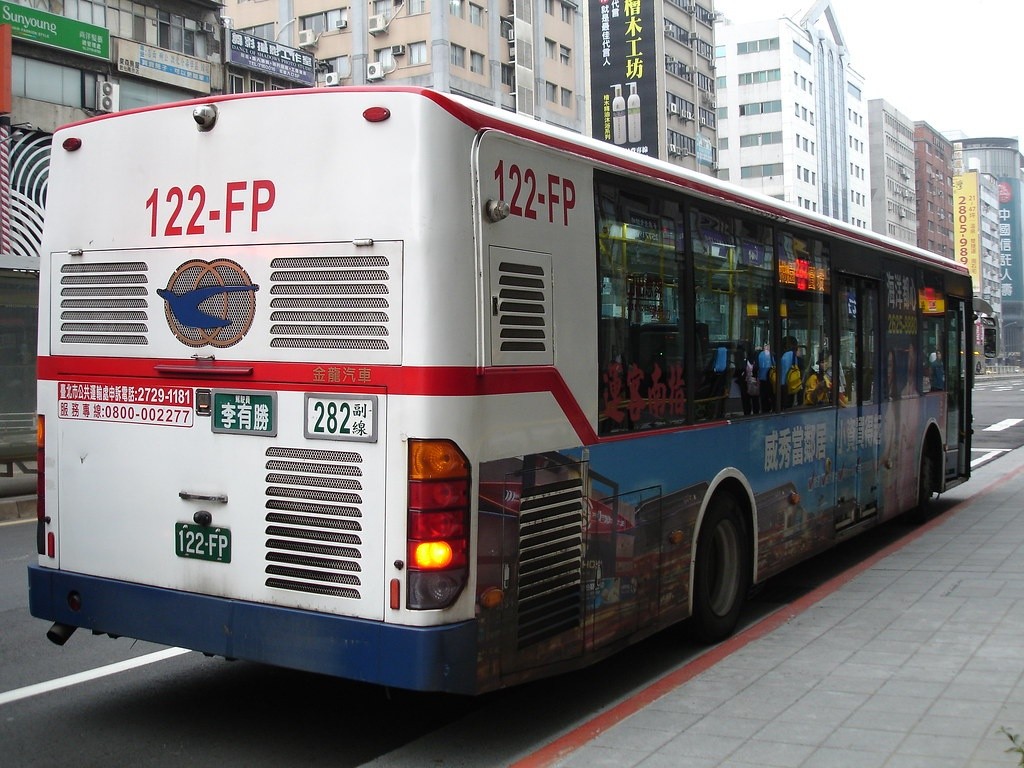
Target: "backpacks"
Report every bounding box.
[803,374,829,406]
[786,353,803,394]
[767,366,777,396]
[746,359,761,397]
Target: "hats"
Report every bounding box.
[811,364,820,374]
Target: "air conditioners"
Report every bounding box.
[508,29,513,40]
[676,147,682,157]
[664,24,675,33]
[668,144,676,154]
[707,12,717,21]
[196,22,213,33]
[509,48,514,56]
[687,6,696,14]
[699,117,705,126]
[95,81,120,113]
[685,64,697,73]
[685,111,691,122]
[390,45,404,55]
[712,162,719,171]
[930,172,946,221]
[368,13,387,33]
[682,148,688,157]
[325,72,339,85]
[710,101,716,109]
[688,32,699,40]
[666,56,678,63]
[709,59,715,67]
[298,29,316,48]
[366,61,383,80]
[681,110,687,119]
[702,91,710,101]
[670,103,676,114]
[335,19,346,29]
[897,167,915,219]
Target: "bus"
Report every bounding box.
[26,85,998,698]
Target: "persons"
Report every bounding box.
[886,348,899,518]
[803,348,849,409]
[703,335,805,419]
[929,350,946,393]
[899,338,921,514]
